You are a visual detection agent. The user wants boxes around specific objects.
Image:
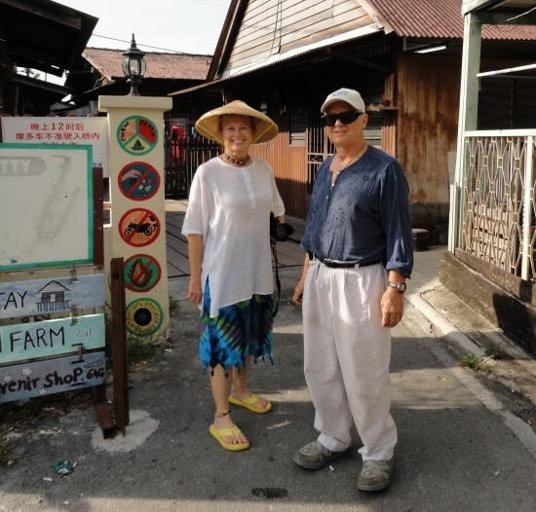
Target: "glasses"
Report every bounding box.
[320,112,364,126]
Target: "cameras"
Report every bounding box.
[270,211,294,242]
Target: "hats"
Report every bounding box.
[319,87,366,115]
[194,99,280,147]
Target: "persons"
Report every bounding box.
[181,98,288,450]
[292,88,414,493]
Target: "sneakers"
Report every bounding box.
[356,444,395,494]
[291,439,351,471]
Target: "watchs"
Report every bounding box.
[389,280,407,294]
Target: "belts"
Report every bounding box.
[315,256,385,270]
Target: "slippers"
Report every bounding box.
[226,390,273,415]
[208,420,251,453]
